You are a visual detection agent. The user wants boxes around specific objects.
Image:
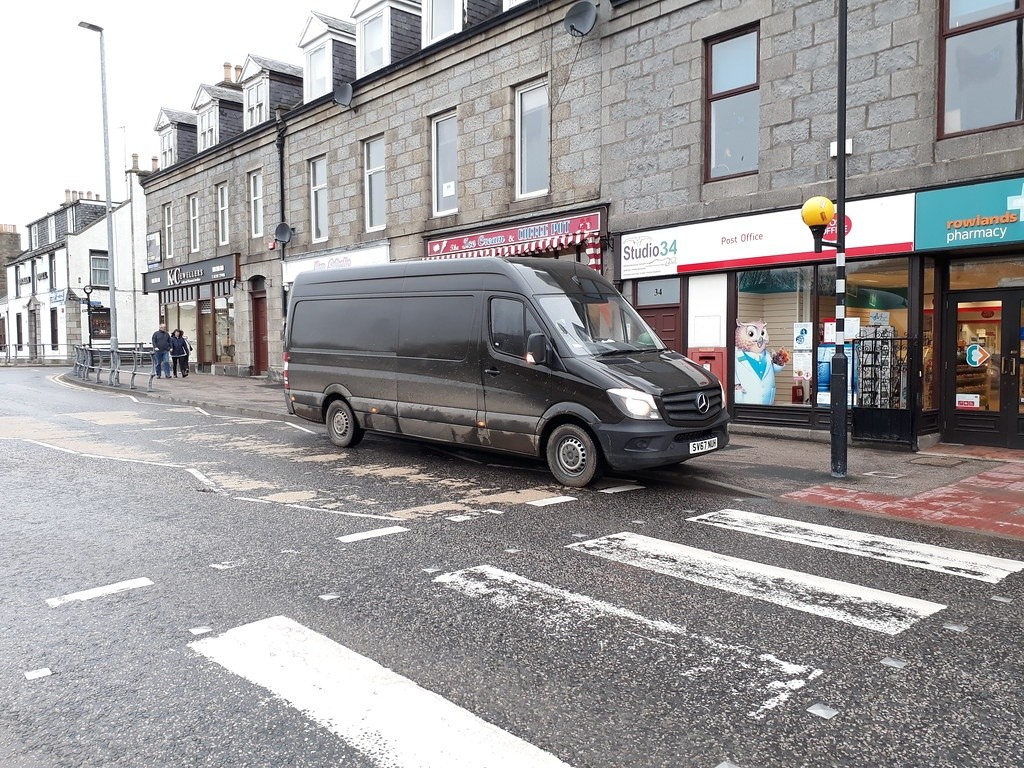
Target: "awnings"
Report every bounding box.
[422,231,601,273]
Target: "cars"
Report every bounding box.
[850,288,1000,408]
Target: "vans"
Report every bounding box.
[281,254,731,489]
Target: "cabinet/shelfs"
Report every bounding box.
[955,357,991,409]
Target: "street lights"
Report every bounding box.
[78,21,121,384]
[83,285,94,372]
[800,196,849,478]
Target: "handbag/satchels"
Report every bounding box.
[190,346,193,351]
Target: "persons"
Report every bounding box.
[152,324,192,379]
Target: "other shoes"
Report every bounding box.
[157,375,160,379]
[182,373,188,377]
[174,374,177,378]
[165,375,172,378]
[185,369,189,373]
